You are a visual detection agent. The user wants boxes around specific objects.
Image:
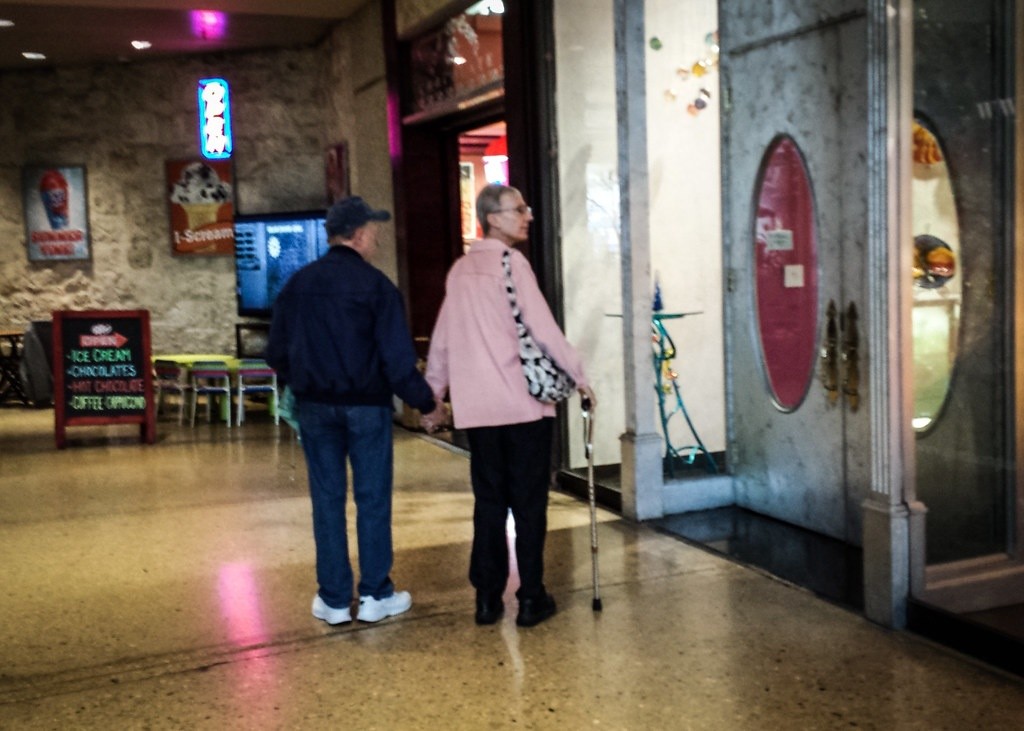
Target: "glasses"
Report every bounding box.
[492,204,532,215]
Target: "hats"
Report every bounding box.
[912,234,955,287]
[326,197,391,235]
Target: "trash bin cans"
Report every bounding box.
[22,318,53,405]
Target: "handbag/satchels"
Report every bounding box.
[518,329,576,405]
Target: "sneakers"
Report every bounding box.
[356,590,412,622]
[312,594,352,624]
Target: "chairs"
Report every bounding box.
[154,360,280,427]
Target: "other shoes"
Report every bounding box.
[516,592,557,627]
[475,597,504,625]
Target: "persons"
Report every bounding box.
[263,198,449,625]
[417,184,598,627]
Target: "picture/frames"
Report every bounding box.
[20,164,91,263]
[164,155,241,259]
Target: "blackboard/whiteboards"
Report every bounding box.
[52,310,155,426]
